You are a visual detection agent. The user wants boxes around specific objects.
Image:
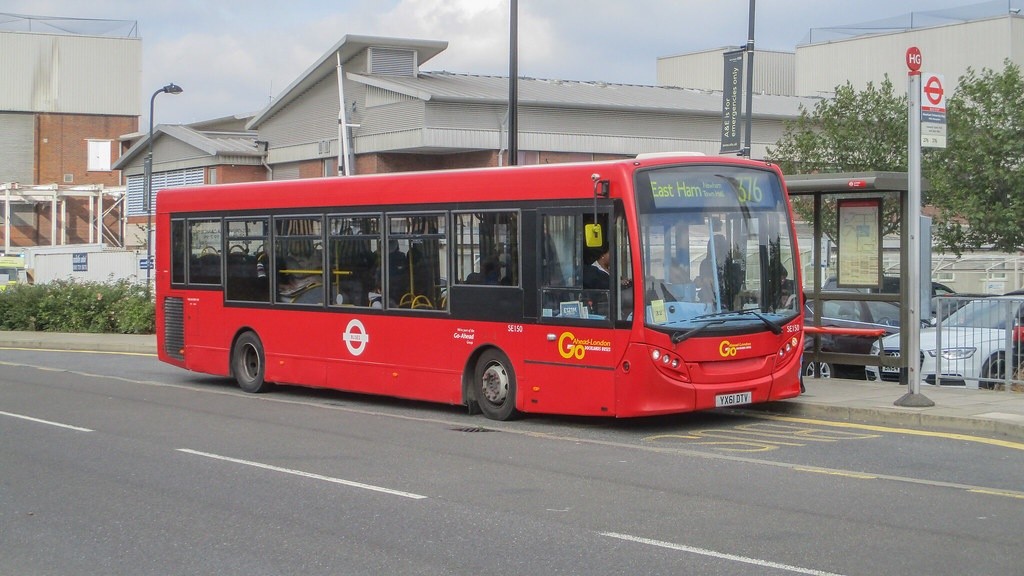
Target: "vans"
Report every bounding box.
[823,272,955,300]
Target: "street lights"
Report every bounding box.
[145,84,183,301]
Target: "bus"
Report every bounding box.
[155,151,804,419]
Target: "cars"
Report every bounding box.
[865,294,1024,390]
[930,291,1000,322]
[803,294,934,378]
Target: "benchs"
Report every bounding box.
[805,327,886,362]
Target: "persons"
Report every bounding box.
[698,233,736,310]
[256,226,429,309]
[779,263,807,393]
[582,242,630,315]
[466,255,503,285]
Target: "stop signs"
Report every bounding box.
[920,73,946,112]
